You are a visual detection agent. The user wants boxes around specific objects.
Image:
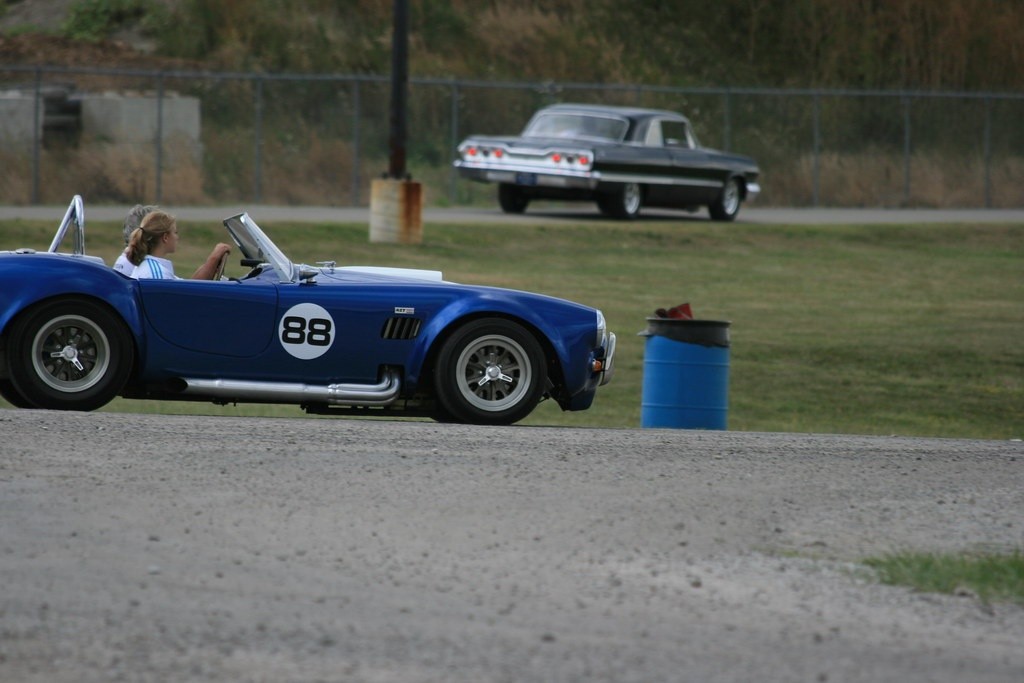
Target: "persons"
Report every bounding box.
[111,204,232,281]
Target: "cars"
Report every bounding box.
[0,194,616,426]
[453,102,761,221]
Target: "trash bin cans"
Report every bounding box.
[368,178,426,245]
[640,317,733,429]
